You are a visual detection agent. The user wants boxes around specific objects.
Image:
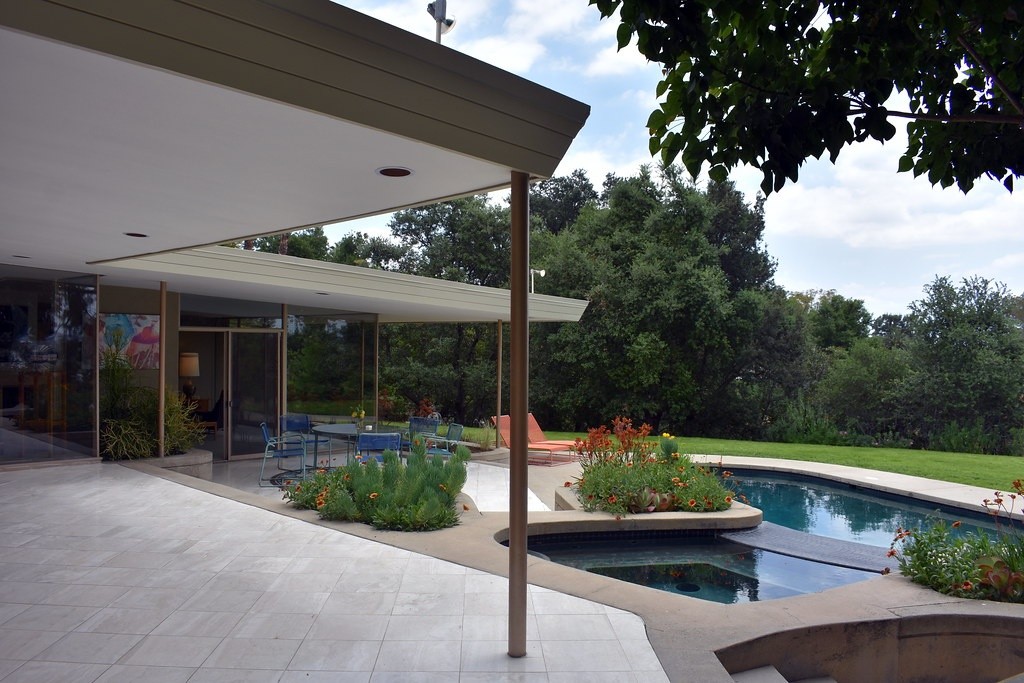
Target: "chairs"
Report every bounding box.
[257,413,463,487]
[527,412,590,462]
[491,415,572,466]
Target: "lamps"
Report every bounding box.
[179,352,201,406]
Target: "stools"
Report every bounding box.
[191,411,218,439]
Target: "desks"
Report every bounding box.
[311,423,406,471]
